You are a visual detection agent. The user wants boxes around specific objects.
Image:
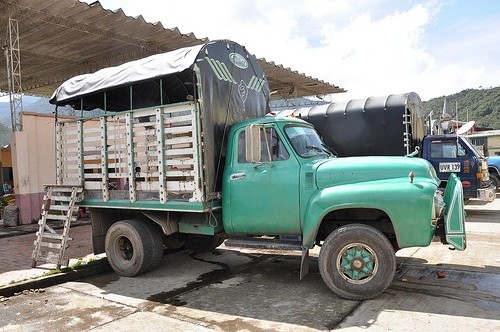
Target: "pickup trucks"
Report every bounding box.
[487,155,500,188]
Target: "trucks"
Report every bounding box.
[55,44,468,301]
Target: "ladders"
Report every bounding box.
[30,184,84,270]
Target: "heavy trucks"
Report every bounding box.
[272,92,497,202]
